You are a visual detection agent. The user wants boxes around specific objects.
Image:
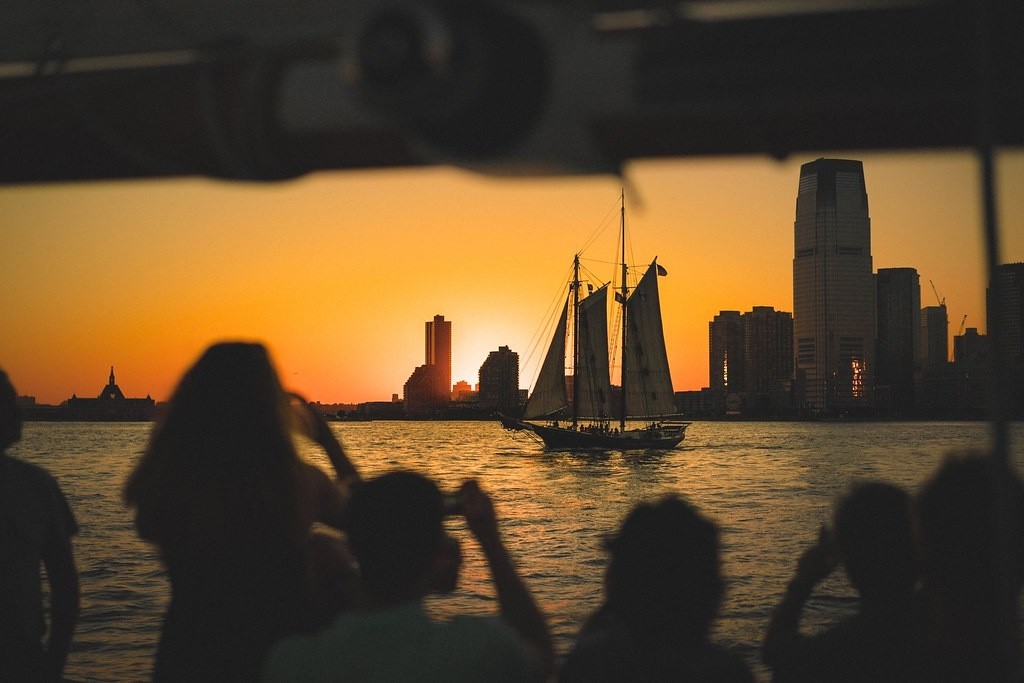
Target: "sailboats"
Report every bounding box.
[495,183,691,451]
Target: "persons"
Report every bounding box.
[122,339,556,683]
[765,456,1023,683]
[562,495,757,683]
[0,370,80,683]
[553,420,662,438]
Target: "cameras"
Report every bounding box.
[440,491,463,516]
